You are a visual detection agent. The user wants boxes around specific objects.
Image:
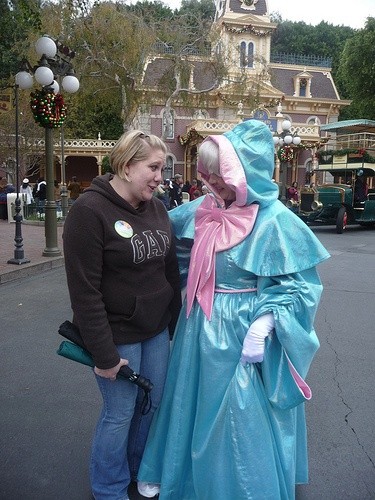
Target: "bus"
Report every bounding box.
[285,119,375,235]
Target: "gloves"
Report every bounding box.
[242,312,275,365]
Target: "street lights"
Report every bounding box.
[272,120,301,206]
[0,69,37,265]
[13,34,80,257]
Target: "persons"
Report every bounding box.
[0,173,301,217]
[165,118,316,500]
[63,131,183,500]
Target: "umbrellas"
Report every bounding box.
[56,320,155,390]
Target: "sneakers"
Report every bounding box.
[137,482,160,498]
